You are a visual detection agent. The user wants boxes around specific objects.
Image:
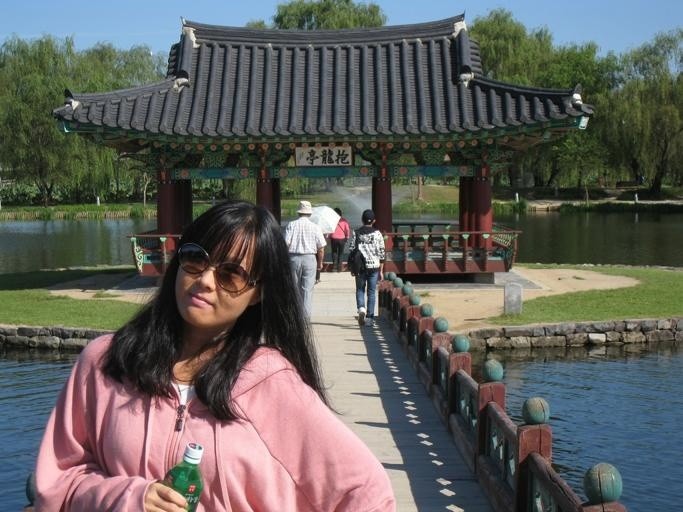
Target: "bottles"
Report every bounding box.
[163,443,204,511]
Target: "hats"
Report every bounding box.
[296,201,312,214]
[363,209,374,220]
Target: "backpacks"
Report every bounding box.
[348,231,365,276]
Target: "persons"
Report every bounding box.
[285,200,350,319]
[348,209,386,327]
[34,199,397,512]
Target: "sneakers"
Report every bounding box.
[358,309,376,327]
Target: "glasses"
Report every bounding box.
[177,242,261,292]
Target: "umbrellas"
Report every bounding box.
[309,205,341,234]
